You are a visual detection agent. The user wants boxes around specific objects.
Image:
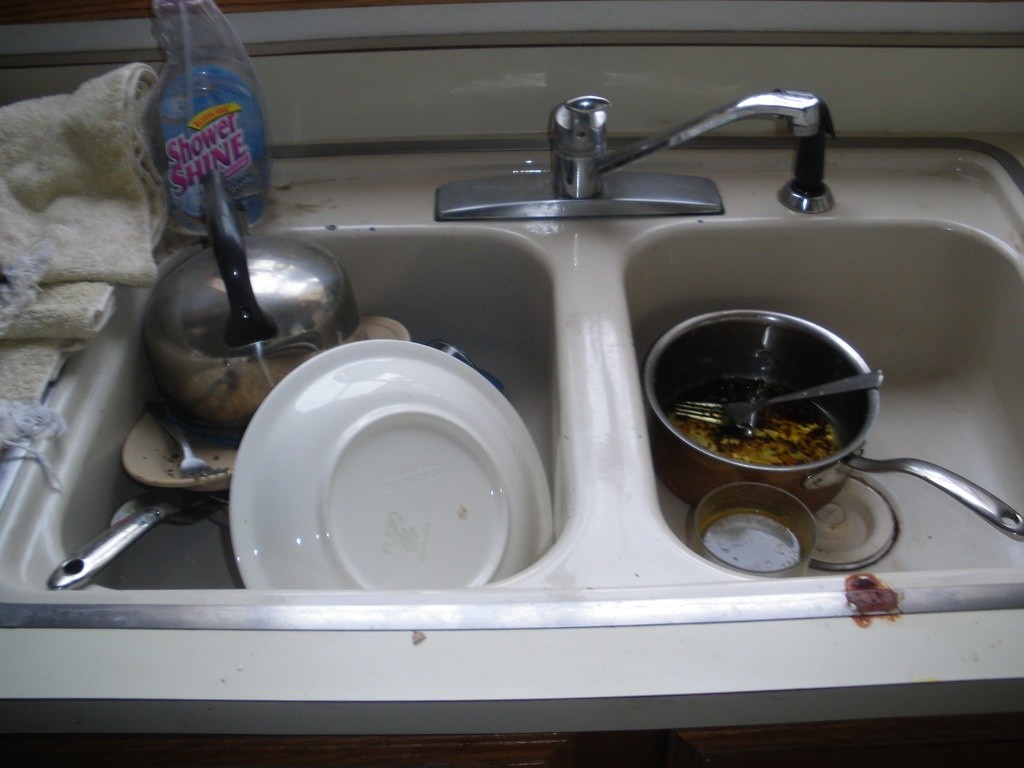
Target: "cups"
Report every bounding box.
[691,479,818,578]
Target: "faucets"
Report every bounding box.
[544,88,822,223]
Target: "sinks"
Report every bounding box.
[615,212,1024,580]
[40,216,579,599]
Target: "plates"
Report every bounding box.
[227,340,554,592]
[121,400,238,493]
[363,317,412,341]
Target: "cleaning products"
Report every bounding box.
[140,0,273,238]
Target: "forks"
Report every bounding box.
[674,372,884,427]
[148,407,230,483]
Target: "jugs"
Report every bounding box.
[140,168,361,444]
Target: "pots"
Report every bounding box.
[642,309,1024,542]
